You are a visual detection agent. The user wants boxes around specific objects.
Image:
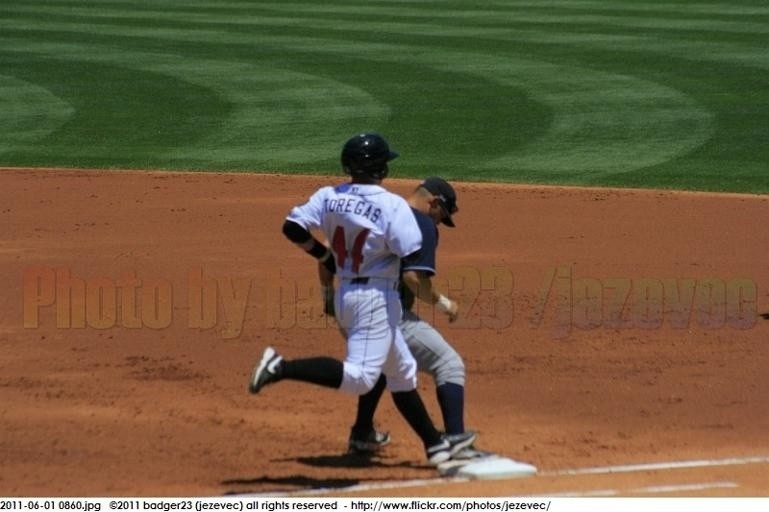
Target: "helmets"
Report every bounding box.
[342,133,400,173]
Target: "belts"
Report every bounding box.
[350,277,369,284]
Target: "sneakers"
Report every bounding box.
[348,430,392,456]
[424,430,475,467]
[438,445,499,478]
[249,345,284,392]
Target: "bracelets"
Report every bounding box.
[433,293,452,313]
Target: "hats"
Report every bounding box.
[418,177,458,228]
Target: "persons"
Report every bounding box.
[347,171,497,475]
[247,133,481,467]
